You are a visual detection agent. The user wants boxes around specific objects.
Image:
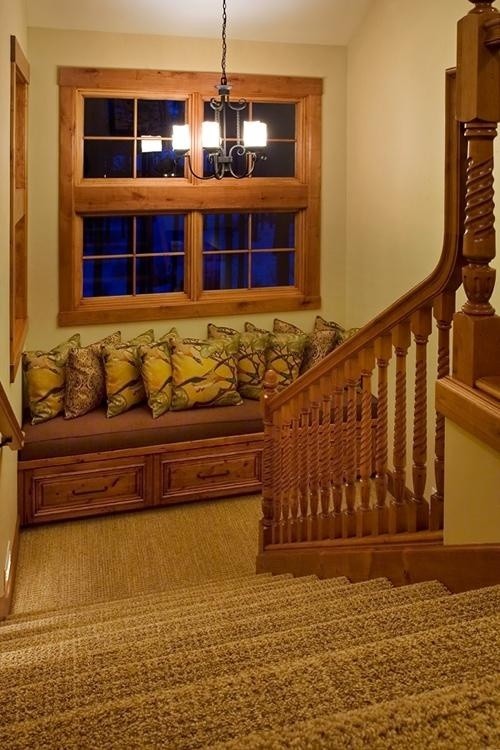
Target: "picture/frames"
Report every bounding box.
[103,99,164,139]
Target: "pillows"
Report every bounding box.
[65,331,122,421]
[136,327,181,420]
[170,337,243,411]
[21,333,80,425]
[207,315,363,402]
[100,329,155,418]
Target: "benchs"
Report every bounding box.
[19,387,382,532]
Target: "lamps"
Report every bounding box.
[169,0,272,182]
[138,134,163,154]
